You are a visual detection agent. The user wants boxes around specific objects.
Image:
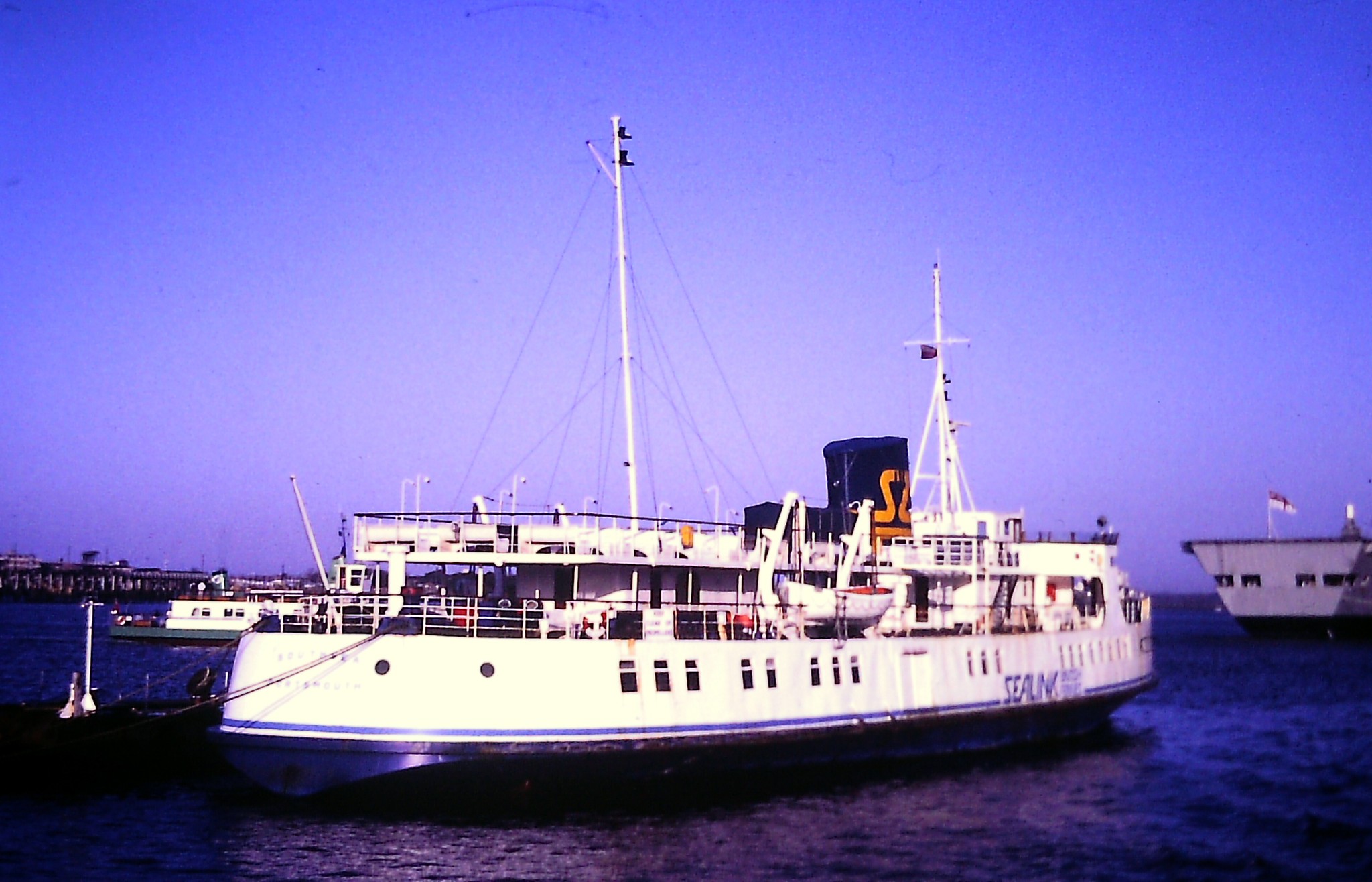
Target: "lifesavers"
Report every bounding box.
[582,609,607,638]
[187,668,215,694]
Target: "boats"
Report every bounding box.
[103,111,1161,809]
[1179,503,1372,640]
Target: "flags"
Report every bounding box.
[1269,491,1296,515]
